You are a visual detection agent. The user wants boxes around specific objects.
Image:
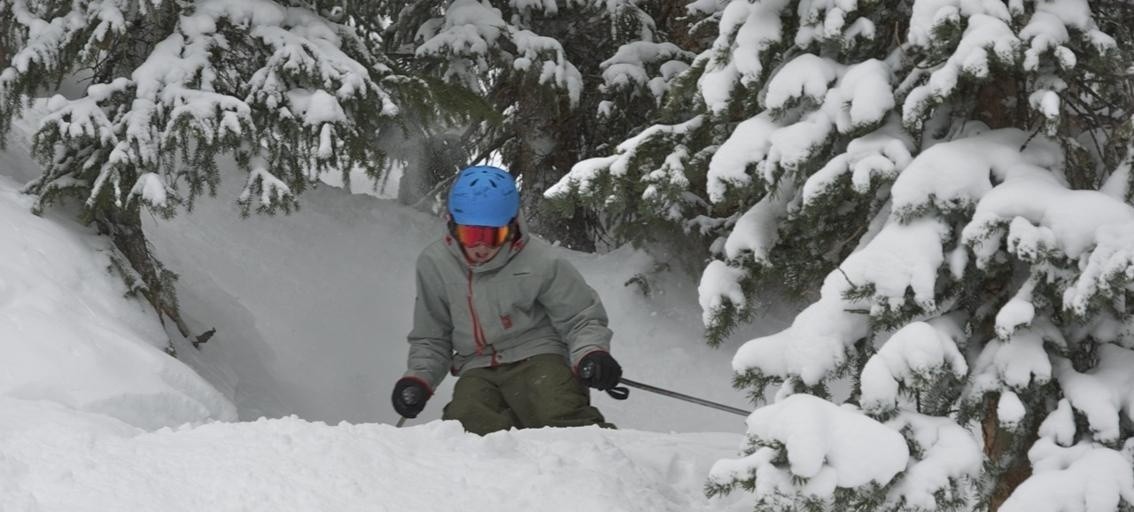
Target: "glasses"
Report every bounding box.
[448,223,510,249]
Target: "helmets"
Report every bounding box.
[447,165,521,229]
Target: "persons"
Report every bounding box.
[389,166,622,437]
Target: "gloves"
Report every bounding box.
[391,377,433,420]
[576,350,623,391]
